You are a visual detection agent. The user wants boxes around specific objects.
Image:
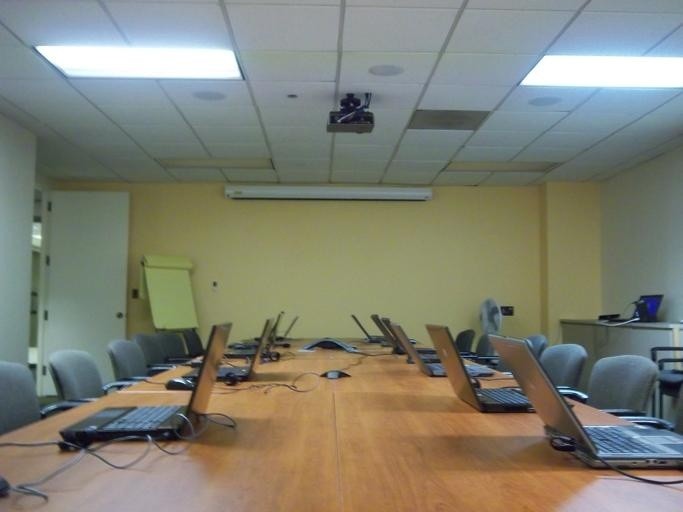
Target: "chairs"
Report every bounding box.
[490,335,547,374]
[614,384,683,439]
[454,330,475,355]
[48,350,138,402]
[135,334,182,367]
[183,330,207,357]
[460,333,494,364]
[109,340,175,383]
[159,330,194,362]
[0,362,83,436]
[558,353,657,416]
[540,344,586,394]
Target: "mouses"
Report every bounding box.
[165,377,194,390]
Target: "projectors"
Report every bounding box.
[326,107,375,134]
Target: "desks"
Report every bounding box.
[0,339,683,511]
[560,320,683,424]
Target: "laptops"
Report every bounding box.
[370,313,436,354]
[351,314,384,343]
[488,332,683,469]
[182,317,273,383]
[58,321,233,444]
[382,317,441,362]
[255,306,297,340]
[424,322,574,412]
[611,294,664,324]
[390,322,496,378]
[222,310,284,358]
